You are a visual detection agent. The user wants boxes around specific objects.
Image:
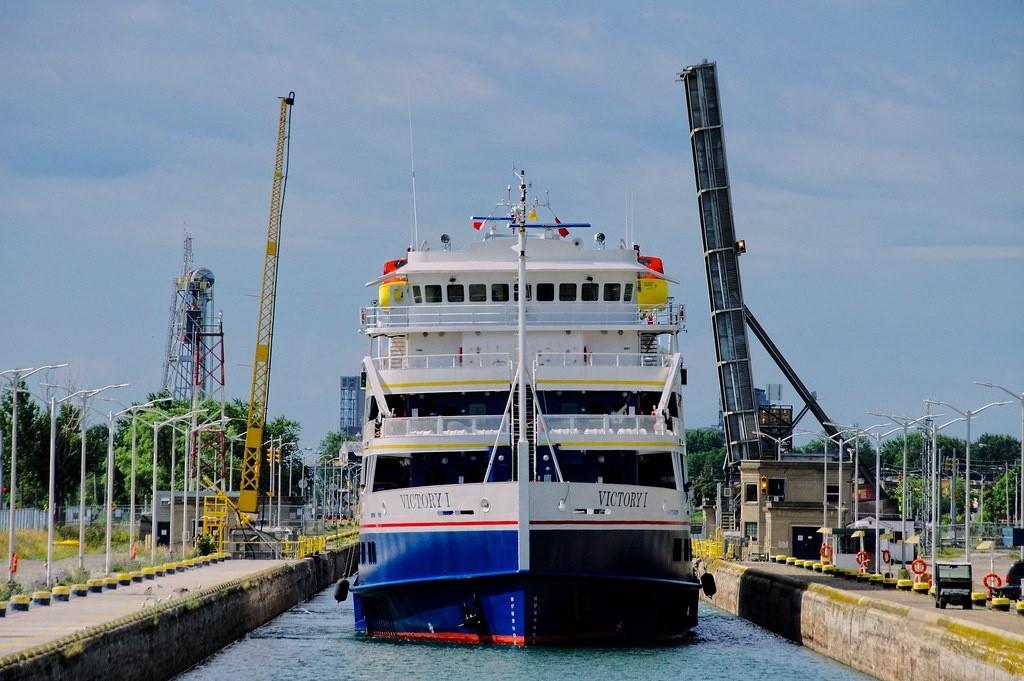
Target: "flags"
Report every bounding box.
[555,217,570,238]
[528,209,538,221]
[474,222,482,229]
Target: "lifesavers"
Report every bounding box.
[911,559,927,574]
[12,552,18,572]
[855,551,871,565]
[819,545,833,558]
[982,573,1002,590]
[132,544,136,558]
[882,549,891,563]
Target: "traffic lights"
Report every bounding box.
[761,475,767,494]
[275,448,280,463]
[266,447,272,463]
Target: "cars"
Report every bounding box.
[935,562,972,609]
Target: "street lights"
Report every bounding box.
[751,431,815,461]
[38,382,129,576]
[924,399,1014,563]
[794,399,976,584]
[0,364,69,583]
[103,394,233,566]
[223,429,361,537]
[974,381,1024,561]
[6,386,98,591]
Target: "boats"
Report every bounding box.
[347,162,706,648]
[378,96,425,309]
[620,187,669,309]
